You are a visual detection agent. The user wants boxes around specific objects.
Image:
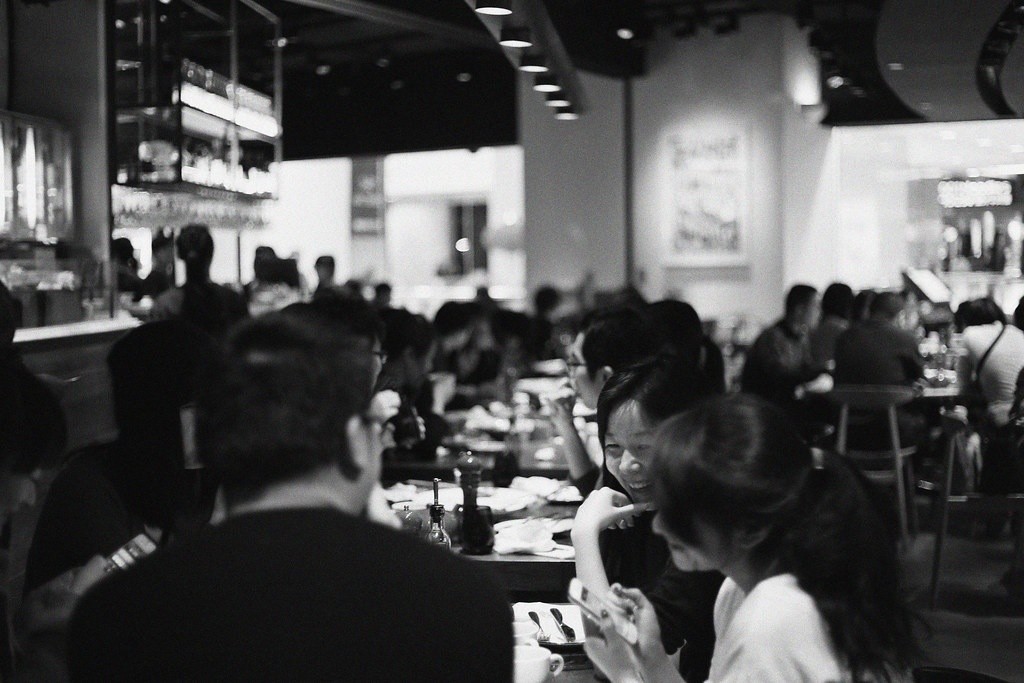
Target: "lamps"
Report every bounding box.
[473,0,578,121]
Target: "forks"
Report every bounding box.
[528,611,549,640]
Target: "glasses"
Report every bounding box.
[370,350,388,365]
[565,357,591,372]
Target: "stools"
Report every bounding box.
[827,386,1024,604]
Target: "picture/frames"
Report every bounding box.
[658,119,755,269]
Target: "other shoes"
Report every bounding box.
[916,475,934,491]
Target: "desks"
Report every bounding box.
[5,360,689,683]
[804,367,969,523]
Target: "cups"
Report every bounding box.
[514,645,563,683]
[454,504,494,554]
[512,620,540,647]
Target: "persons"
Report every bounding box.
[0,283,68,474]
[115,227,560,482]
[545,300,726,683]
[68,312,516,683]
[580,396,928,682]
[741,282,1024,493]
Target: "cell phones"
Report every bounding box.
[568,578,638,645]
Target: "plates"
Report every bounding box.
[514,604,586,645]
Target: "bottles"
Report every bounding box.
[396,505,423,534]
[426,506,451,548]
